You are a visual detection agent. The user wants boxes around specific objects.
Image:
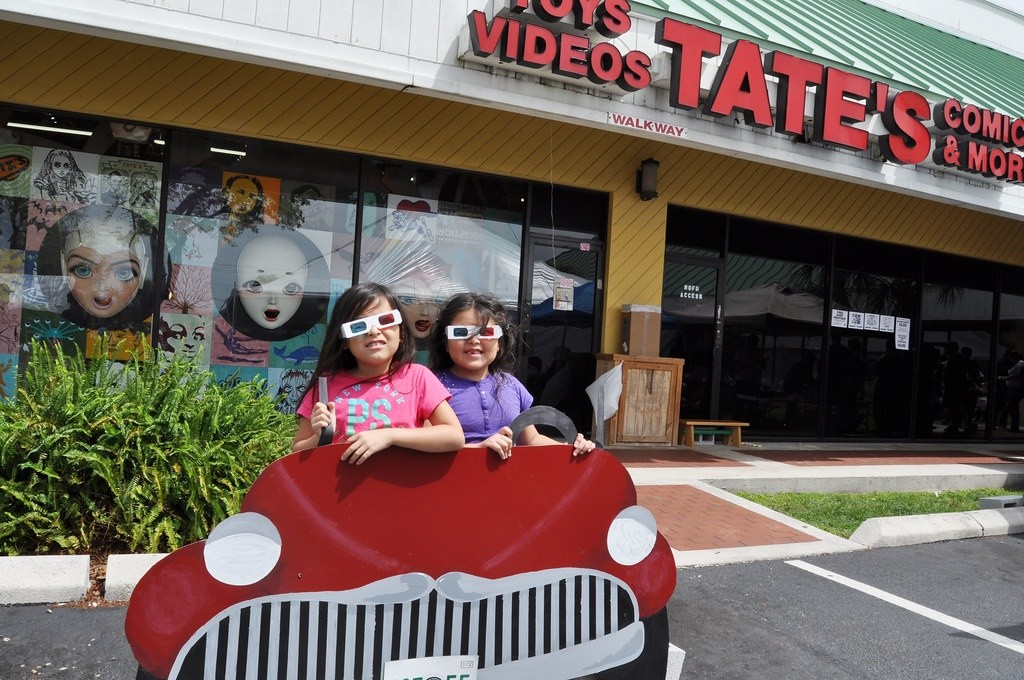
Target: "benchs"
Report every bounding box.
[678,418,750,447]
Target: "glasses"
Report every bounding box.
[445,324,504,340]
[341,308,403,339]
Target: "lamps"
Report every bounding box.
[635,156,661,199]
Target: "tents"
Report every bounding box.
[667,280,872,433]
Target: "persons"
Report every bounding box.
[787,351,820,387]
[828,331,905,434]
[995,350,1024,431]
[728,335,766,420]
[430,292,596,461]
[293,283,464,465]
[943,342,979,431]
